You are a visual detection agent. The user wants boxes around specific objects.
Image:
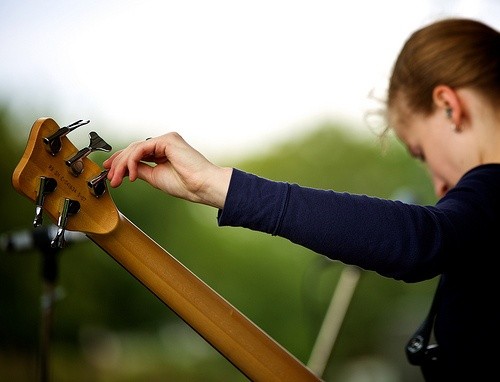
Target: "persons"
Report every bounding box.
[105,17,500,382]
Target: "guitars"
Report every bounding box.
[9,115,327,381]
